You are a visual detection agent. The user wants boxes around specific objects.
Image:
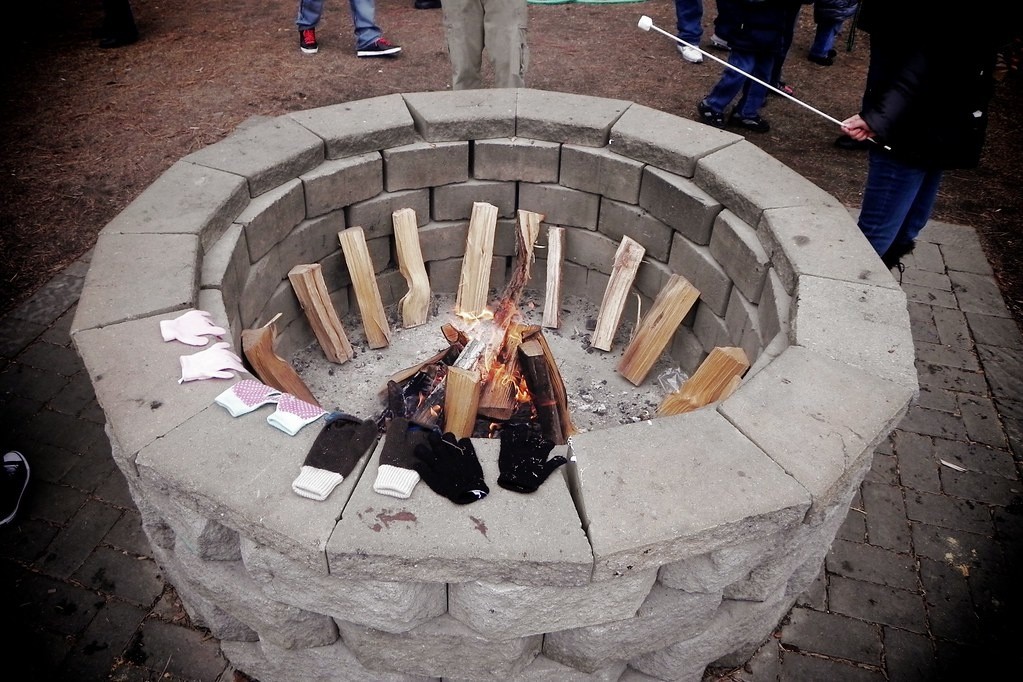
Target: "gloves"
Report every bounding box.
[159,309,227,347]
[179,341,249,384]
[411,431,491,506]
[495,424,569,494]
[293,408,379,503]
[215,375,331,436]
[374,417,431,501]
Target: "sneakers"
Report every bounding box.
[299,27,318,54]
[697,101,725,129]
[0,451,30,524]
[710,33,732,51]
[729,110,772,134]
[358,39,403,56]
[677,41,704,62]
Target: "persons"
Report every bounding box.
[0,450,30,526]
[807,0,859,66]
[675,0,803,134]
[440,0,530,91]
[834,0,1007,286]
[295,0,401,56]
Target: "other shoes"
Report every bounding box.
[809,49,838,66]
